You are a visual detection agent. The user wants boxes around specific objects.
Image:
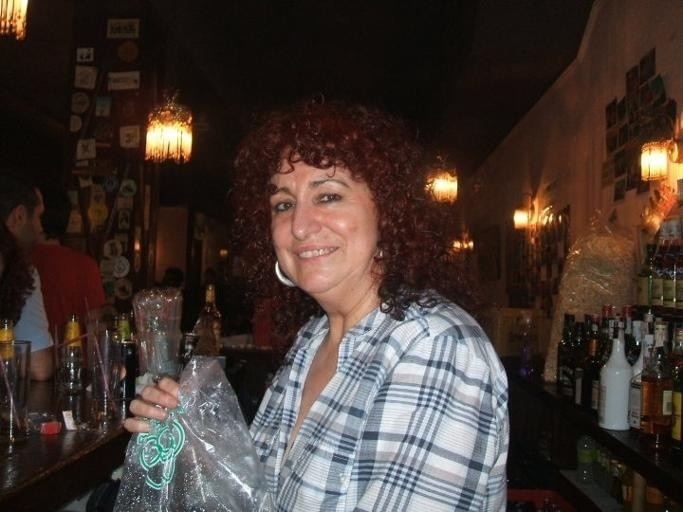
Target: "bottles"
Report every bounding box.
[63,313,82,346]
[112,316,119,338]
[1,320,14,359]
[202,284,221,355]
[118,313,131,342]
[558,217,682,510]
[520,318,534,376]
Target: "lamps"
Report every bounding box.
[640,137,671,180]
[512,206,530,230]
[452,231,474,253]
[145,90,194,162]
[0,0,29,40]
[423,155,458,204]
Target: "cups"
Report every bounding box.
[181,333,200,361]
[1,332,124,443]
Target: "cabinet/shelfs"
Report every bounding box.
[499,306,682,512]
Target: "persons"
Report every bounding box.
[124,97,511,512]
[1,162,105,383]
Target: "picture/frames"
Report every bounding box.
[102,15,145,42]
